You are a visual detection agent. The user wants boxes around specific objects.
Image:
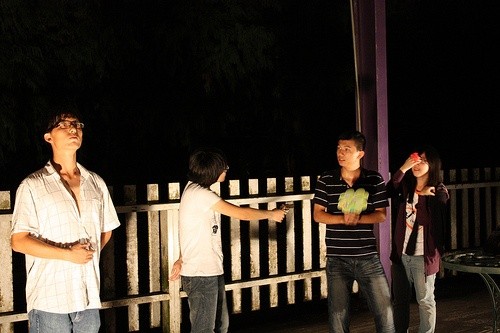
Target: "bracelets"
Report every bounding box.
[359,214,361,224]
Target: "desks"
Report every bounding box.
[441,248,500,333]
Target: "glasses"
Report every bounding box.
[46,117,85,134]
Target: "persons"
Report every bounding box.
[312,131,395,333]
[167,150,289,333]
[10,103,121,333]
[385,143,450,333]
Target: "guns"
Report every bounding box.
[278,200,303,217]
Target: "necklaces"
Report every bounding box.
[416,189,420,192]
